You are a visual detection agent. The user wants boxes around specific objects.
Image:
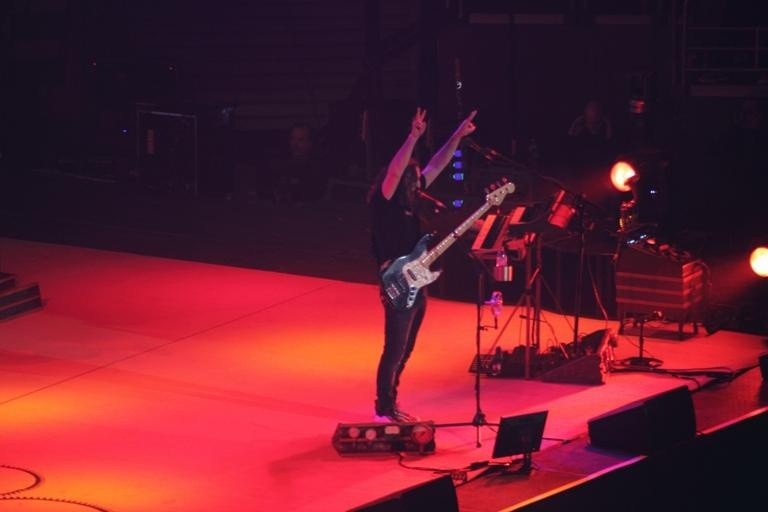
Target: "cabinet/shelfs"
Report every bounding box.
[614,244,705,340]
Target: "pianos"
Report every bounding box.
[468,189,565,262]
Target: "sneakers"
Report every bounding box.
[375,407,409,423]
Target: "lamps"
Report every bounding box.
[612,155,642,194]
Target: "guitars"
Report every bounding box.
[378,177,516,312]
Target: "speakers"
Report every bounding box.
[345,473,460,512]
[588,386,697,456]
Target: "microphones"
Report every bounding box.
[465,137,497,164]
[412,186,445,211]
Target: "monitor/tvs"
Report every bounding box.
[493,410,548,479]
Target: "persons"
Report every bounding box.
[568,100,613,140]
[258,122,329,203]
[366,106,477,426]
